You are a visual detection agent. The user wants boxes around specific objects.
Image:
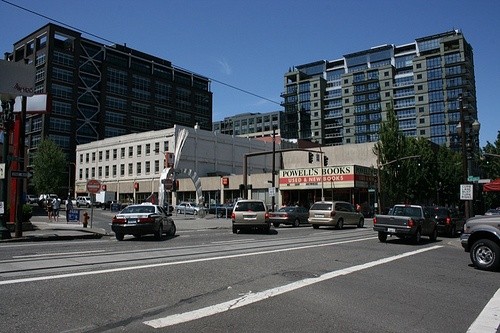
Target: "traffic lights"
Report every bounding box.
[324,156,328,166]
[308,153,313,163]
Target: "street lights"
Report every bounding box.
[456,119,480,218]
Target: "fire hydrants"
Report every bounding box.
[82,212,90,228]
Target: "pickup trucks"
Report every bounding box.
[373,204,440,245]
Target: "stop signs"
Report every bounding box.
[86,180,101,192]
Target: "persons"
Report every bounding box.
[47,199,53,222]
[51,196,61,221]
[65,196,73,211]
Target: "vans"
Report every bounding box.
[76,196,92,208]
[40,194,61,204]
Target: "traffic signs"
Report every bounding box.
[11,171,33,178]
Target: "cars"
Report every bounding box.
[436,206,466,238]
[175,202,209,215]
[308,201,364,230]
[269,206,310,228]
[26,195,39,204]
[460,214,500,271]
[231,200,269,234]
[111,205,176,241]
[209,204,234,218]
